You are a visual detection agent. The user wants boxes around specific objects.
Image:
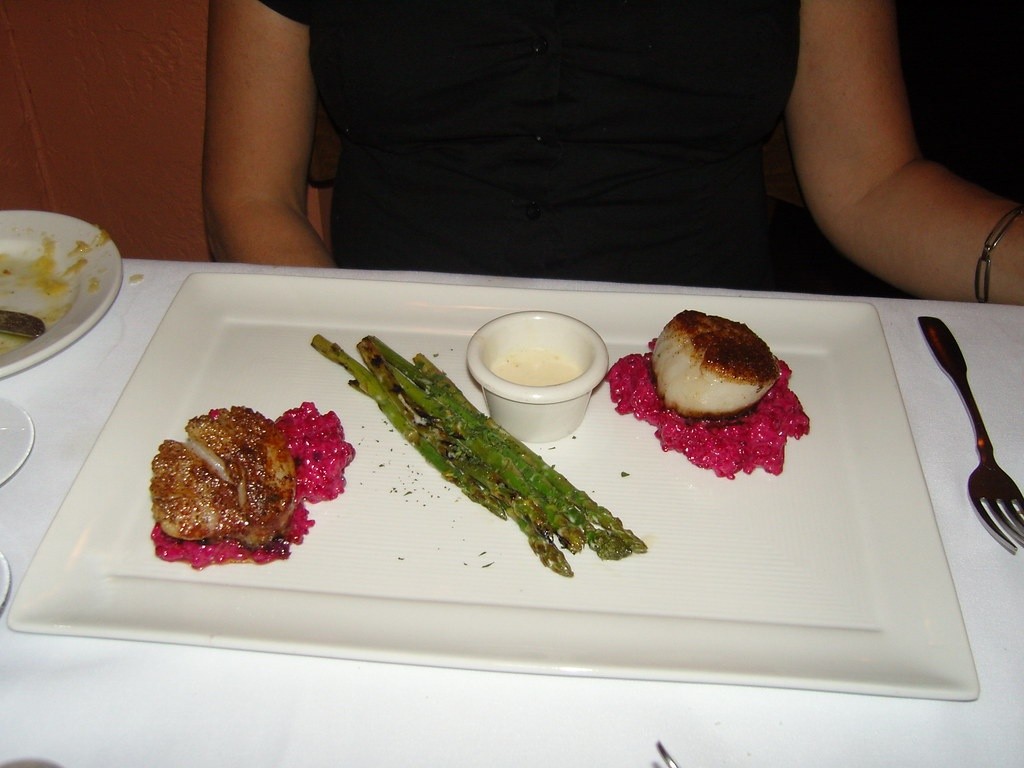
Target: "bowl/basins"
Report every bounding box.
[466,310,609,444]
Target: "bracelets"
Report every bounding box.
[973,204,1023,302]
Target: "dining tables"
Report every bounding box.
[0,258,1024,768]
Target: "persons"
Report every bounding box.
[201,0,1024,308]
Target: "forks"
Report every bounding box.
[11,272,981,702]
[916,316,1024,553]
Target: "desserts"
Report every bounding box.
[651,309,782,418]
[151,405,297,550]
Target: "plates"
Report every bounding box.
[0,210,123,379]
[1,550,11,609]
[0,396,35,485]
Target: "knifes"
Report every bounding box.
[0,310,48,341]
